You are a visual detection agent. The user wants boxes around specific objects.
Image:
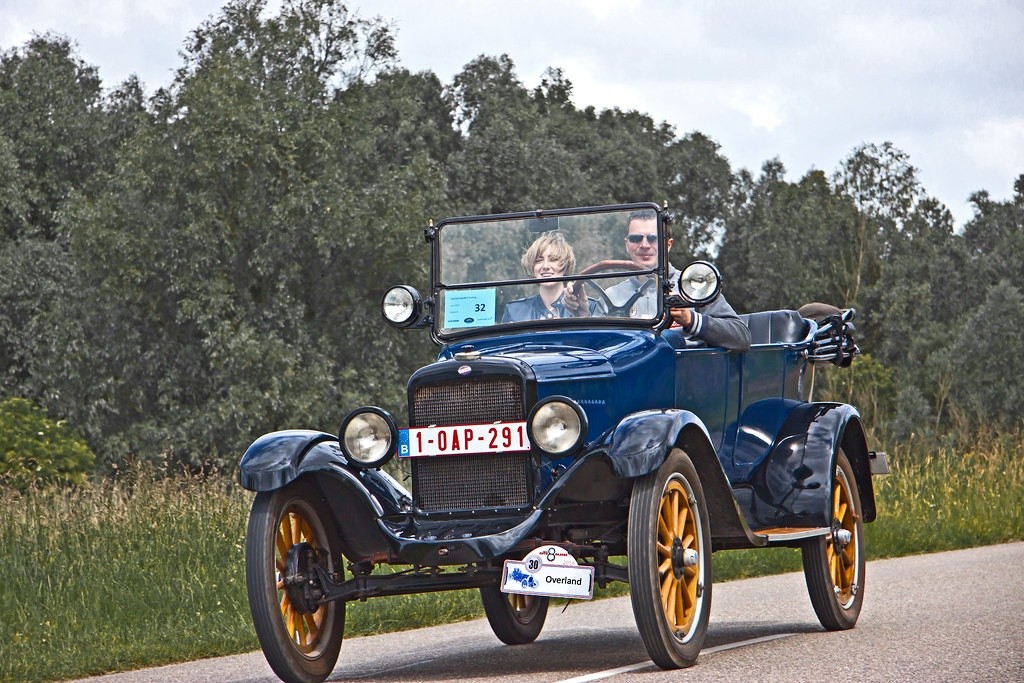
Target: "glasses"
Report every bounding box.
[627,234,658,243]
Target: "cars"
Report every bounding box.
[236,201,888,683]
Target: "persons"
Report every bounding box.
[550,208,751,351]
[500,228,598,323]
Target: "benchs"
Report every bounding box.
[739,309,806,344]
